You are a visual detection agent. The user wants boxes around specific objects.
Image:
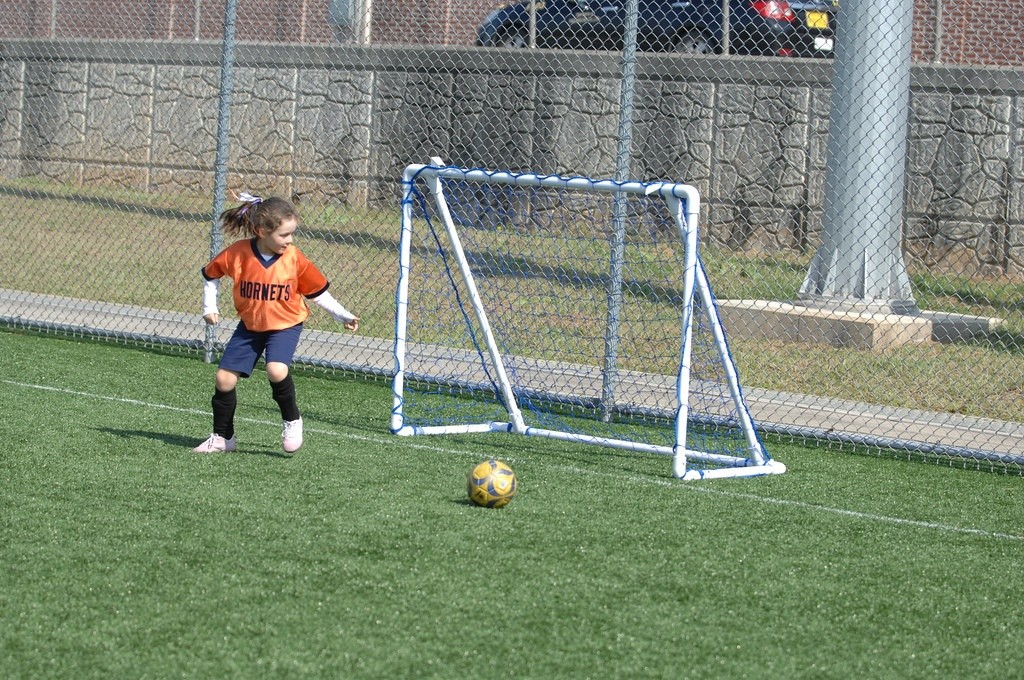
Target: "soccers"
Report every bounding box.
[466,460,518,509]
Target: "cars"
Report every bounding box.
[474,1,835,57]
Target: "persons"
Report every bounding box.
[191,187,361,455]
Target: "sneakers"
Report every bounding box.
[282,415,303,453]
[192,432,238,453]
